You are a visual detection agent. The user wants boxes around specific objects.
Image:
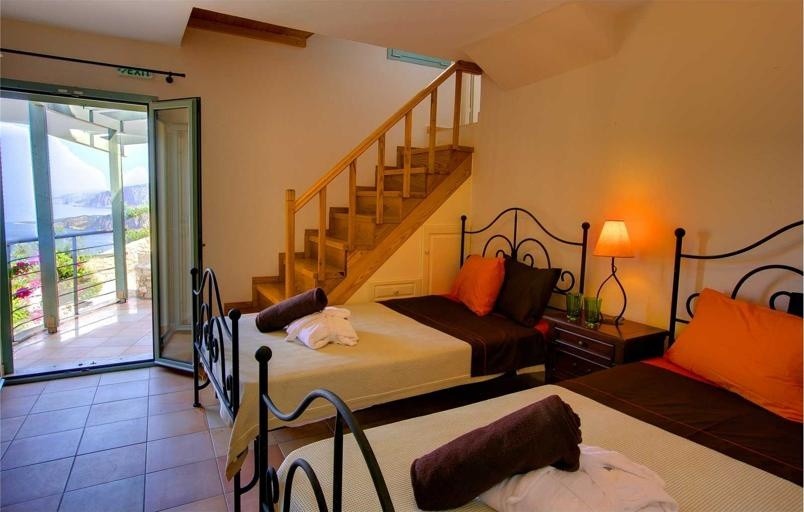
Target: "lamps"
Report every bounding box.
[591,219,637,328]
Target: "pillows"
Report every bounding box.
[499,252,563,328]
[663,288,803,422]
[449,254,506,316]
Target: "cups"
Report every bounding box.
[565,291,601,330]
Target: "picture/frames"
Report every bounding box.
[387,47,452,69]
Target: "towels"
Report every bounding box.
[255,284,360,351]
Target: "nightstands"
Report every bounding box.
[544,309,670,383]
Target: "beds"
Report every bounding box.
[190,206,591,511]
[253,216,804,512]
